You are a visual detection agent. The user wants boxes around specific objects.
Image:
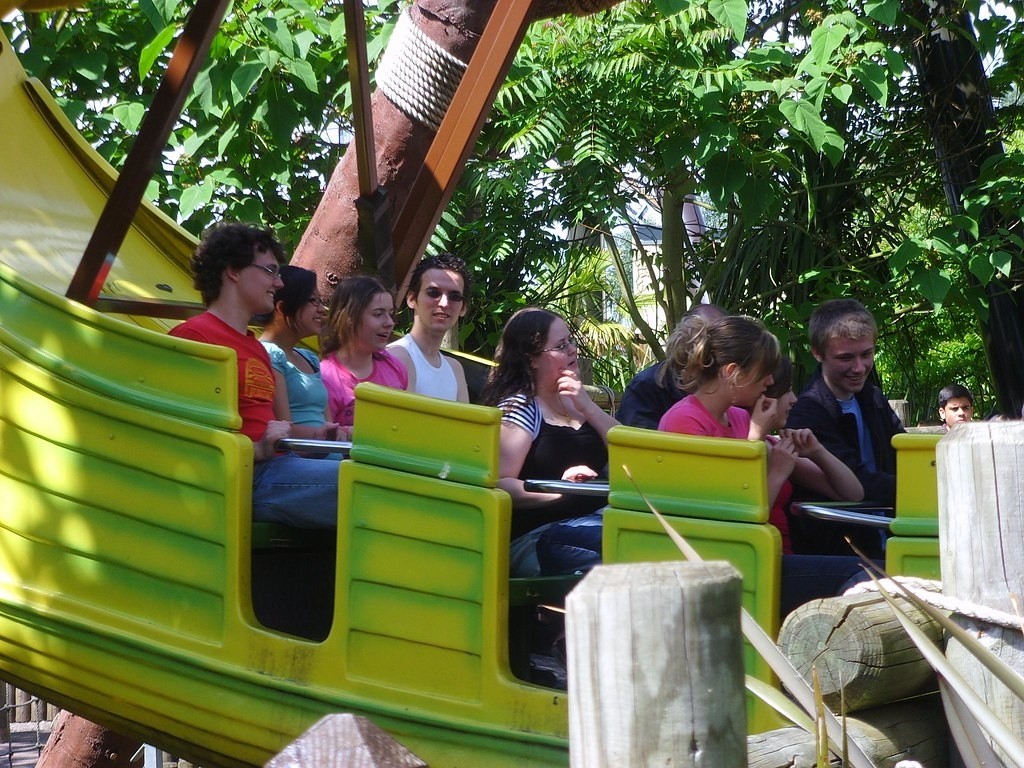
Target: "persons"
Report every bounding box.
[782,297,906,559]
[616,304,725,429]
[169,226,346,527]
[259,266,354,460]
[939,384,974,431]
[383,253,470,404]
[321,277,408,426]
[658,316,885,614]
[482,306,623,671]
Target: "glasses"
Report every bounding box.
[541,337,577,353]
[250,264,281,277]
[420,289,465,302]
[308,297,324,307]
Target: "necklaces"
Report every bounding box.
[542,392,571,422]
